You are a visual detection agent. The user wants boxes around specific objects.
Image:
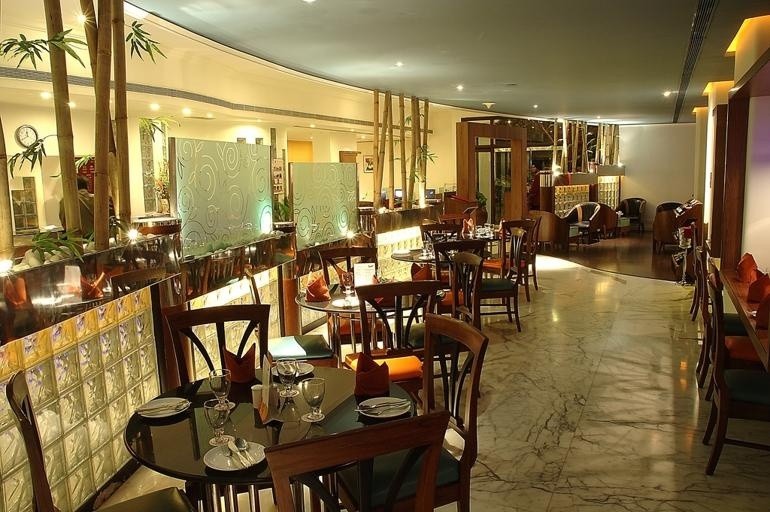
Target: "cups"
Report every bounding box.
[425,244,434,259]
[484,224,492,237]
[276,358,298,397]
[476,225,482,238]
[208,369,235,410]
[204,398,231,444]
[301,377,326,420]
[421,241,430,255]
[490,223,495,237]
[341,272,354,294]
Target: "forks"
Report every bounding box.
[219,443,244,470]
[355,400,406,411]
[139,399,189,411]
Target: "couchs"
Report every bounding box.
[597,202,619,240]
[526,210,566,252]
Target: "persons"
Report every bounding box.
[59,176,95,239]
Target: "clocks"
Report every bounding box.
[16,123,38,150]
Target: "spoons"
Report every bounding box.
[365,401,410,416]
[232,437,257,465]
[141,402,189,416]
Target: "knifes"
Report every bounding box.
[226,440,253,468]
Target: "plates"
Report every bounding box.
[138,396,191,419]
[331,299,360,307]
[203,441,266,472]
[357,397,412,418]
[270,362,315,376]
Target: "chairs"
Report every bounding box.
[562,202,602,245]
[164,303,278,506]
[614,198,647,233]
[343,279,442,414]
[470,226,528,333]
[263,409,452,511]
[693,262,753,376]
[492,214,543,291]
[432,238,486,316]
[689,279,770,475]
[651,199,692,255]
[419,223,463,248]
[5,367,198,512]
[690,237,737,322]
[394,251,485,399]
[316,246,392,358]
[437,212,470,224]
[321,313,490,511]
